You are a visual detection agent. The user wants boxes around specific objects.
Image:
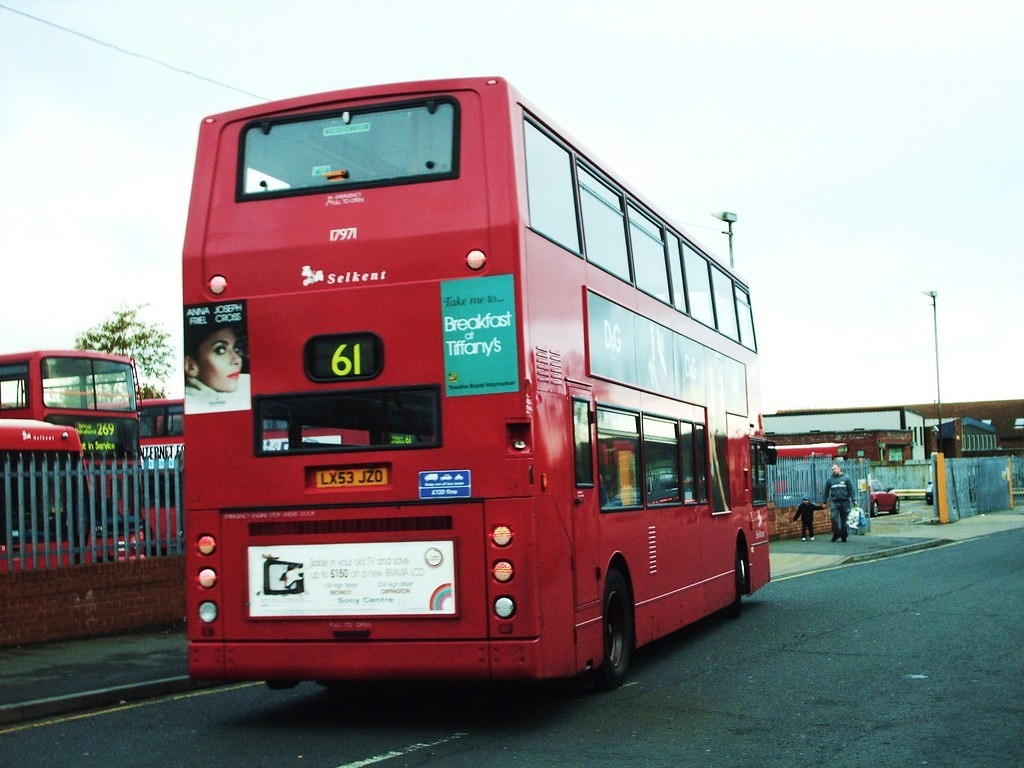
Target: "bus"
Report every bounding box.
[2,350,147,563]
[181,75,778,693]
[769,442,850,494]
[0,419,98,571]
[83,398,183,554]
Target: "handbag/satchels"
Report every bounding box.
[845,505,859,529]
[855,503,866,528]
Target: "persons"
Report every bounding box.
[791,465,857,542]
[184,322,252,415]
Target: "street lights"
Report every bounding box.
[711,212,736,270]
[924,289,944,453]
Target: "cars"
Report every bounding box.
[857,479,901,518]
[775,491,823,508]
[926,482,934,505]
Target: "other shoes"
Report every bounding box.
[841,536,847,542]
[831,533,841,542]
[802,537,805,541]
[810,536,814,540]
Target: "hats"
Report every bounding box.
[802,494,810,501]
